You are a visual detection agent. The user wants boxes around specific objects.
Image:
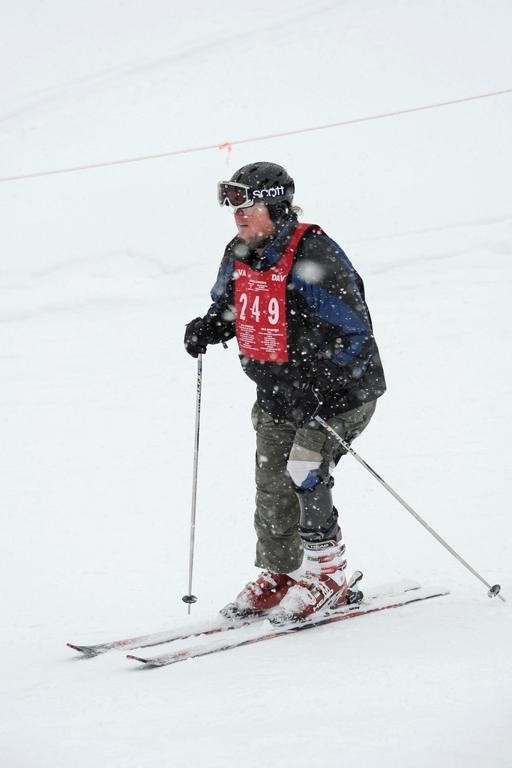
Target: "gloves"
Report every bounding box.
[182,310,231,358]
[293,374,340,420]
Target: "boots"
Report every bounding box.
[267,528,348,627]
[217,568,297,619]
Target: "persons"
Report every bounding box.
[186,162,388,627]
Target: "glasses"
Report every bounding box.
[217,181,257,210]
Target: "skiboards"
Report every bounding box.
[65,575,449,667]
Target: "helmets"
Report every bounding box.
[229,160,297,214]
